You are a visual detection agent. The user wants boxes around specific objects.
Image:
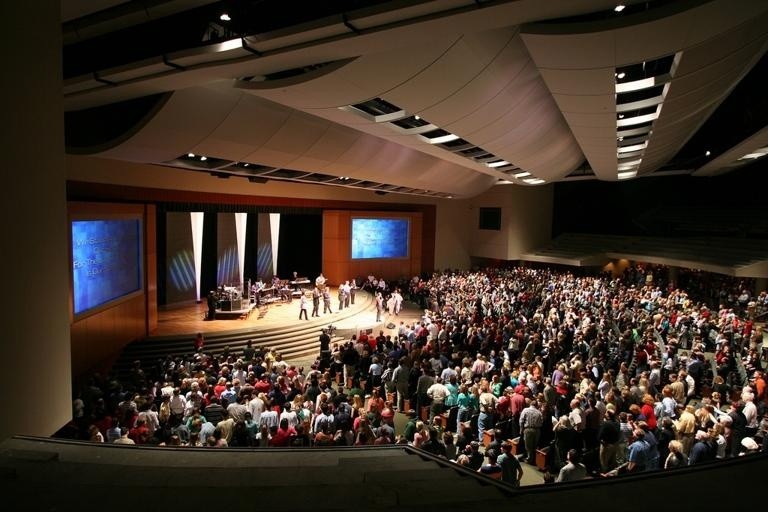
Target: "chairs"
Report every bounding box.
[393,268,767,478]
[66,334,397,450]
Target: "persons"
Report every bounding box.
[220,283,231,300]
[250,278,267,305]
[72,321,411,450]
[207,290,218,321]
[292,272,302,291]
[338,279,361,309]
[299,273,333,321]
[271,274,289,301]
[407,263,767,484]
[360,273,404,321]
